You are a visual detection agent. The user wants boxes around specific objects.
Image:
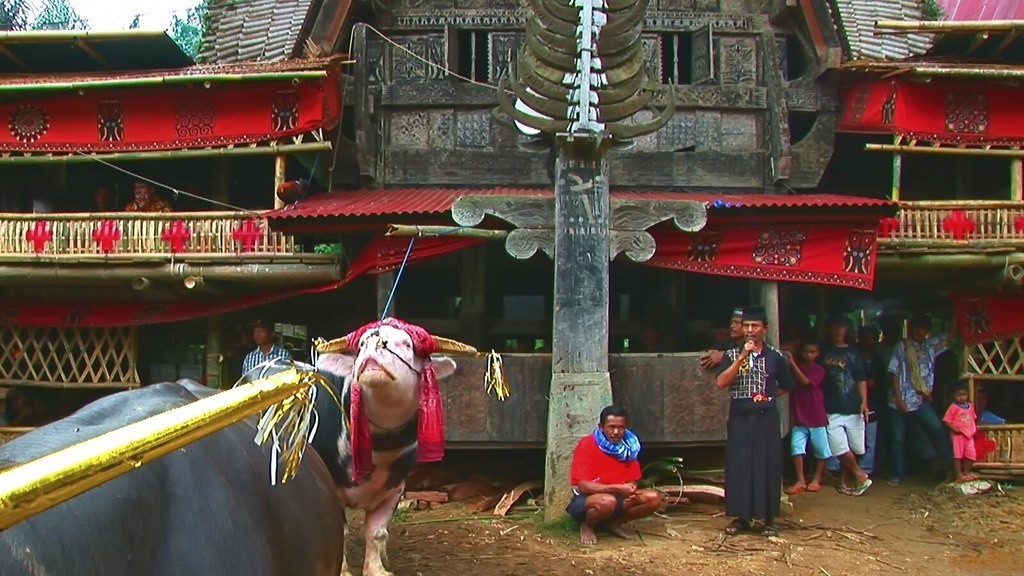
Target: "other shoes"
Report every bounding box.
[954,472,980,482]
[887,476,900,486]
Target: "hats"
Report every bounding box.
[247,317,274,330]
[731,305,744,316]
[742,304,768,324]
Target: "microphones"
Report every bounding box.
[748,340,754,370]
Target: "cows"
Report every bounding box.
[0,319,477,576]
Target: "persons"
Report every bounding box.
[700,302,1017,495]
[565,406,662,545]
[88,174,172,218]
[714,302,796,537]
[242,318,294,386]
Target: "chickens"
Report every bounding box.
[277,174,307,213]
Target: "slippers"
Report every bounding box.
[724,519,755,534]
[806,482,822,491]
[760,523,780,535]
[853,479,873,496]
[784,484,807,494]
[837,485,852,495]
